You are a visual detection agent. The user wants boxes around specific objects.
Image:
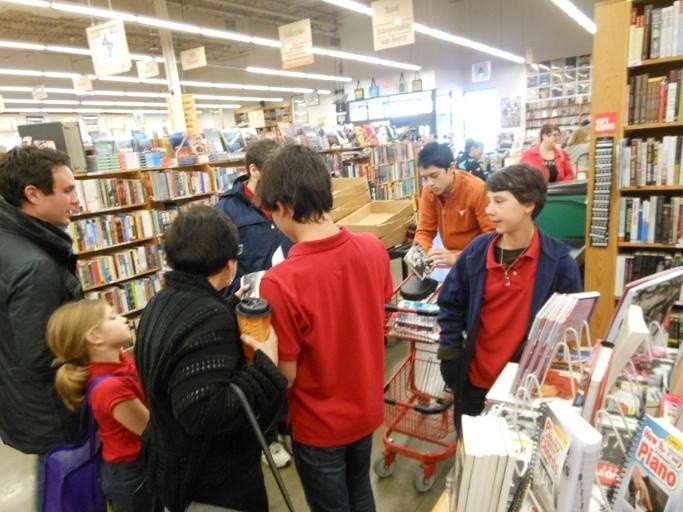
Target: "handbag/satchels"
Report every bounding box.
[41,427,103,511]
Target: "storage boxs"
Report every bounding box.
[330,176,418,251]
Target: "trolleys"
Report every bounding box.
[374,245,458,493]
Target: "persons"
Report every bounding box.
[411,142,496,266]
[519,124,577,185]
[0,143,85,512]
[437,163,582,436]
[253,143,394,512]
[455,138,487,180]
[45,299,150,511]
[136,201,288,511]
[213,138,295,471]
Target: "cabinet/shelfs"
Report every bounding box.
[232,103,293,135]
[523,94,591,150]
[614,1,683,348]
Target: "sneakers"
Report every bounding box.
[262,441,293,469]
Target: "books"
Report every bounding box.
[65,140,441,349]
[451,252,683,510]
[614,1,683,250]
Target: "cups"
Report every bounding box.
[235,298,271,361]
